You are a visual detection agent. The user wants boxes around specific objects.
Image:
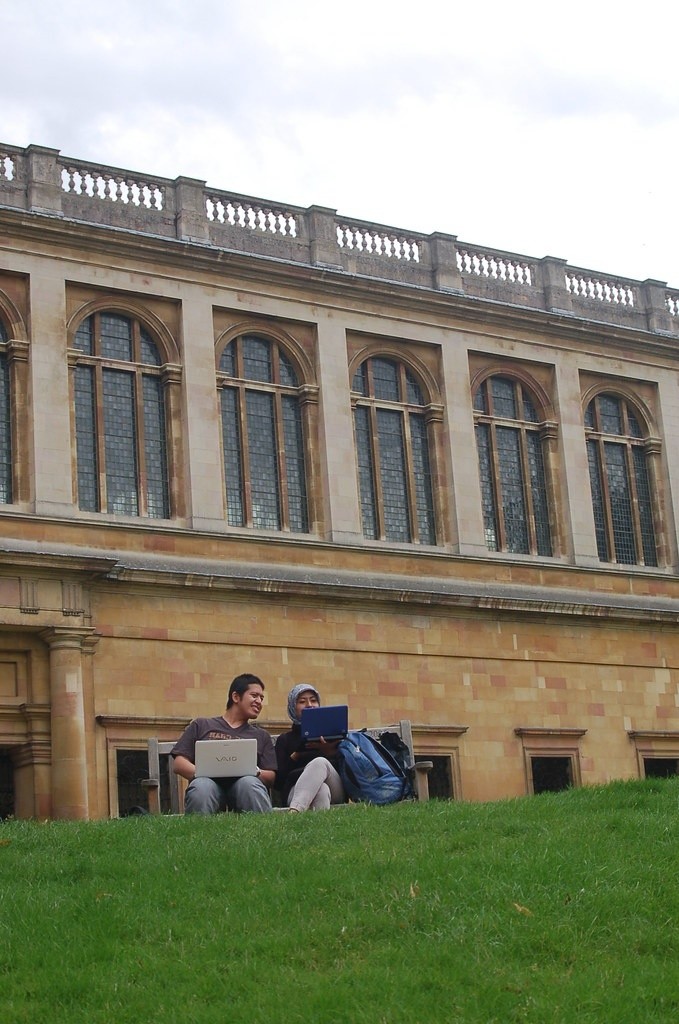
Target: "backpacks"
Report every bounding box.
[336,731,412,806]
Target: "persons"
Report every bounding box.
[275,683,345,813]
[169,673,278,815]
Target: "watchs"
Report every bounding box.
[255,766,261,778]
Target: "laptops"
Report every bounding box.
[296,704,348,753]
[194,738,257,778]
